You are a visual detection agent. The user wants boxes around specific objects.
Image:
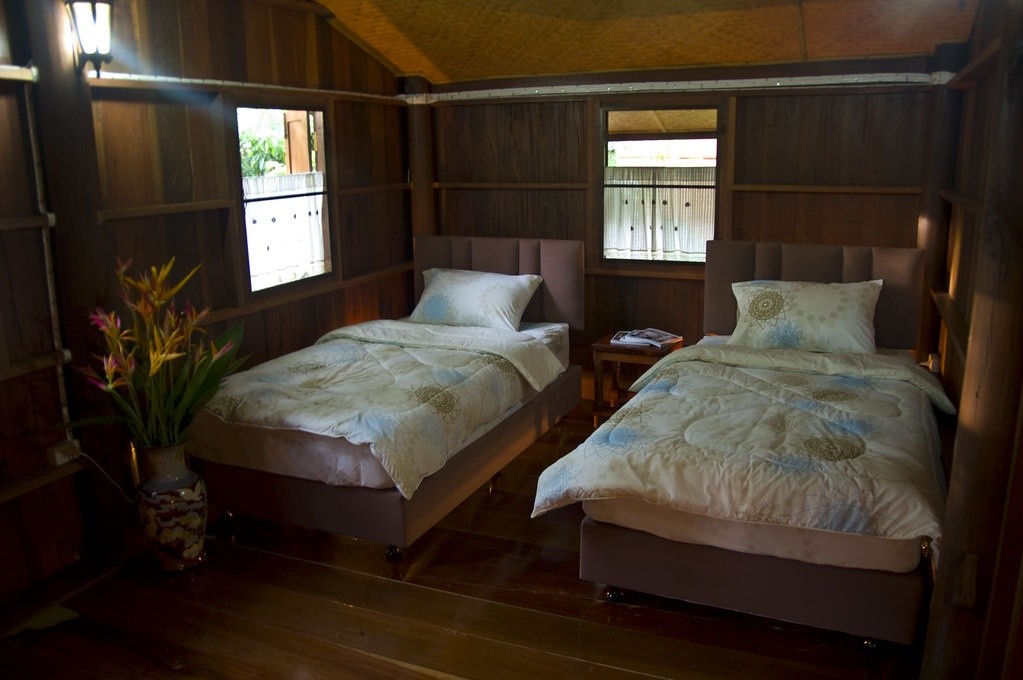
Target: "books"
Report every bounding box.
[611,328,684,347]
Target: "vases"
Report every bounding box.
[129,446,209,572]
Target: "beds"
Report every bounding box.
[531,240,956,652]
[182,235,585,561]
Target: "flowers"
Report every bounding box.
[71,256,253,447]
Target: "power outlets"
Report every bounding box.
[42,437,82,466]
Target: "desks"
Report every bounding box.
[592,335,687,429]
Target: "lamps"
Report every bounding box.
[64,0,112,80]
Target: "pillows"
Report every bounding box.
[409,267,543,332]
[726,278,883,356]
